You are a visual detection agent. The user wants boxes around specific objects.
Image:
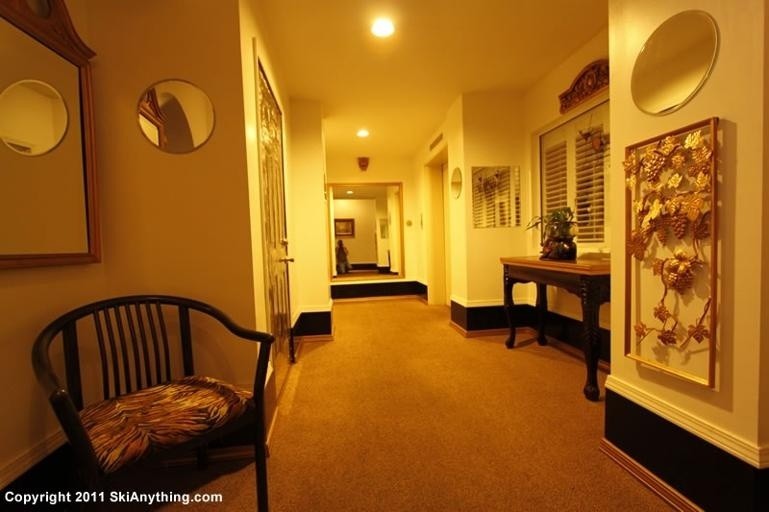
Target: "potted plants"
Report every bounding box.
[525,206,592,260]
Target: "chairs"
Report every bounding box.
[30,290,275,512]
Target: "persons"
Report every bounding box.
[336,240,350,274]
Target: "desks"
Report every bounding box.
[496,253,611,399]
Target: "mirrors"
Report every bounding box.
[0,0,103,268]
[471,165,522,228]
[450,168,461,197]
[136,77,216,155]
[0,79,69,158]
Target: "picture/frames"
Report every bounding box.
[623,119,719,390]
[335,219,356,237]
